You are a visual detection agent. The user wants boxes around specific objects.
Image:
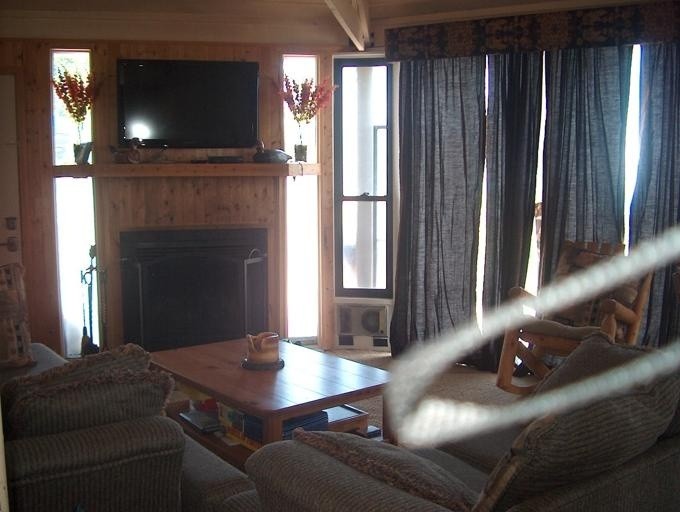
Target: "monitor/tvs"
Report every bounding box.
[117,59,259,147]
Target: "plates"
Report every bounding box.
[207,155,245,164]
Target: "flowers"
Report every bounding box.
[42,51,99,145]
[268,69,335,144]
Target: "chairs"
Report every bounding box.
[492,239,663,398]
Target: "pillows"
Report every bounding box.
[1,260,36,370]
[4,368,173,440]
[2,340,154,407]
[512,326,661,430]
[292,422,478,509]
[474,366,680,510]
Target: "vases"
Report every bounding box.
[71,142,93,166]
[294,142,306,163]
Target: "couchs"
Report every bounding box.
[0,257,258,509]
[225,337,680,510]
